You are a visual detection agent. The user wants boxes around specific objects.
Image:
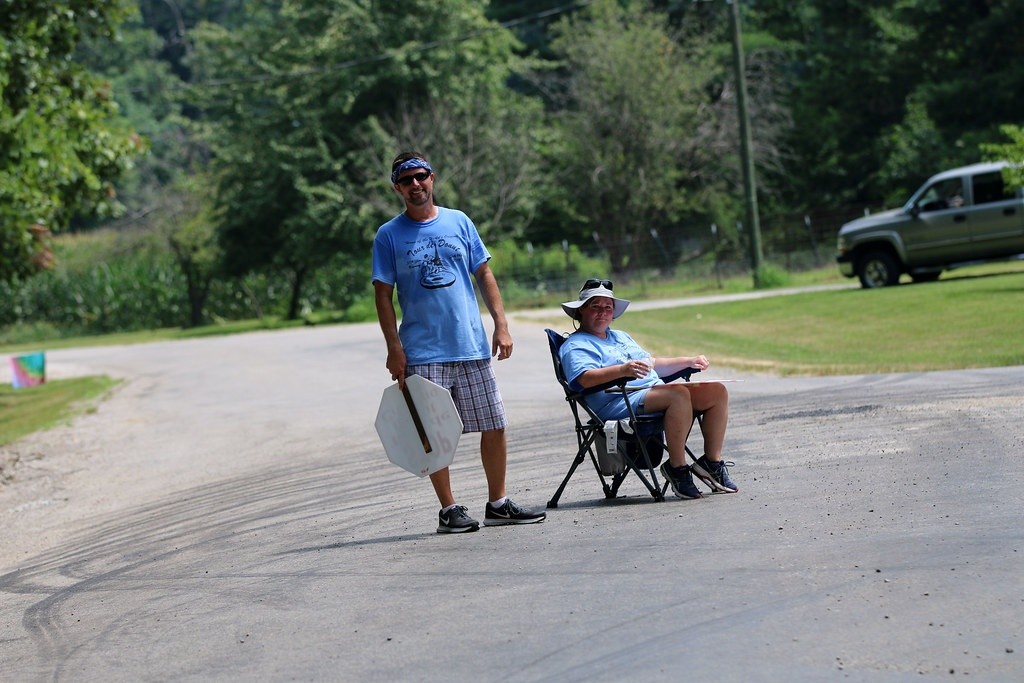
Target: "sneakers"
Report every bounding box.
[437,505,479,533]
[660,458,700,499]
[483,498,546,526]
[692,454,738,493]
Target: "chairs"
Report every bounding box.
[544,328,724,508]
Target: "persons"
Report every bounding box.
[559,278,740,500]
[373,152,547,534]
[944,177,964,206]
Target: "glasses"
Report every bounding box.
[580,280,613,292]
[396,171,430,185]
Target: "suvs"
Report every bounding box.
[836,162,1024,287]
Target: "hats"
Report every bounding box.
[561,285,630,319]
